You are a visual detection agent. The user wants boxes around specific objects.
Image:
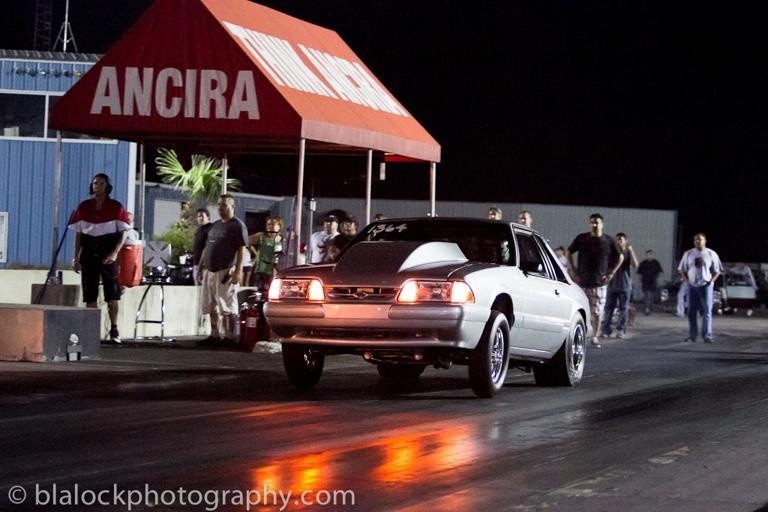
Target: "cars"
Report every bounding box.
[263,217,594,396]
[659,266,768,314]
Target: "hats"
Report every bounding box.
[325,215,336,222]
[345,214,356,222]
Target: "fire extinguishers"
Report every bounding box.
[240,302,259,349]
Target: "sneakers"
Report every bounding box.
[591,337,602,349]
[705,338,714,342]
[685,338,695,342]
[206,336,235,346]
[110,329,122,344]
[616,332,624,339]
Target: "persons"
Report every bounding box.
[64,171,133,345]
[175,194,723,351]
